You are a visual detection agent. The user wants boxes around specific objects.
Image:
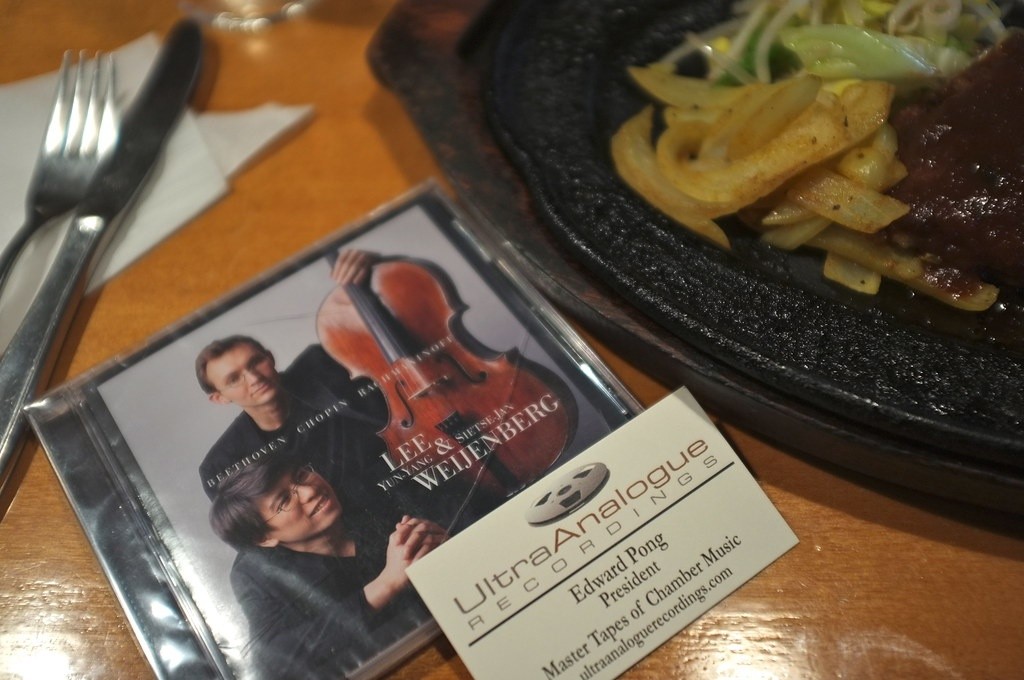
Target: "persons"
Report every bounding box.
[195,248,397,516]
[207,454,449,680]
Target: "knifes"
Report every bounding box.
[0,17,201,492]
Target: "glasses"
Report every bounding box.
[217,352,267,391]
[261,461,315,523]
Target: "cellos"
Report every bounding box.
[314,249,581,501]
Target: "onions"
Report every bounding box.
[609,73,1000,310]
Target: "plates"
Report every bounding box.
[365,0,1024,551]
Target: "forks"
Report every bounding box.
[0,48,120,294]
